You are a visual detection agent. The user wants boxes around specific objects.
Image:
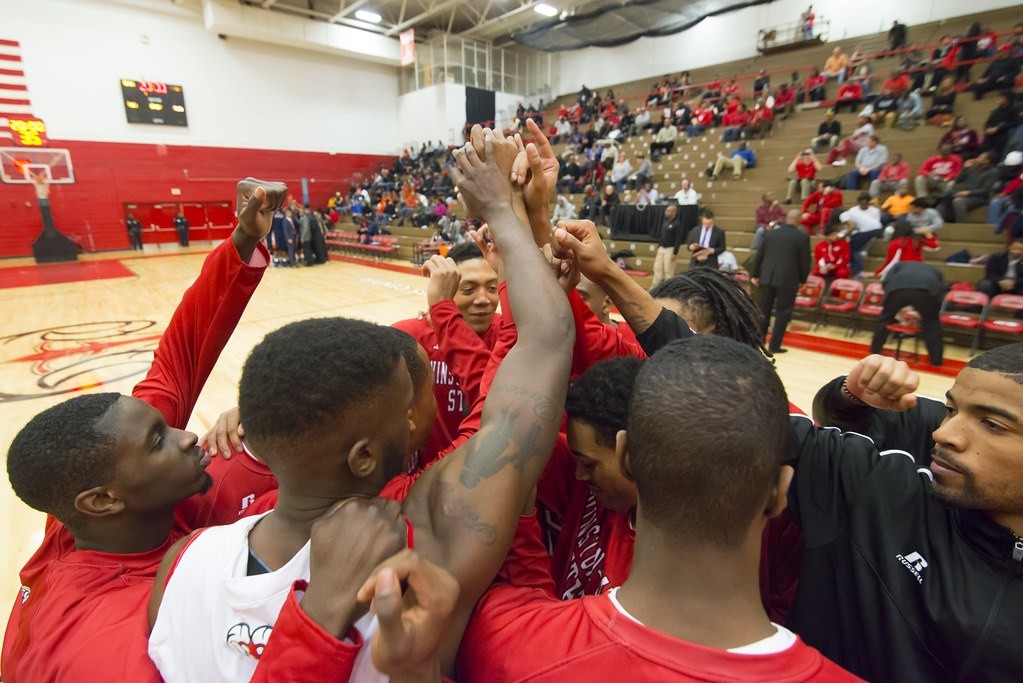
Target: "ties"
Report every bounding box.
[702,228,708,245]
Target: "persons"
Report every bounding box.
[1,5,1023,683]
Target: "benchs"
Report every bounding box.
[332,2,1023,342]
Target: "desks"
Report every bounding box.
[612,202,698,244]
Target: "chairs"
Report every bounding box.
[735,270,1023,362]
[322,230,402,263]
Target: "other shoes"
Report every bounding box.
[782,200,791,204]
[770,345,787,353]
[709,175,716,180]
[832,158,848,167]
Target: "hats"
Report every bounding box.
[1003,151,1022,166]
[825,223,837,235]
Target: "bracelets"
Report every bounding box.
[843,374,868,407]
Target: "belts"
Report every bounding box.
[658,244,673,247]
